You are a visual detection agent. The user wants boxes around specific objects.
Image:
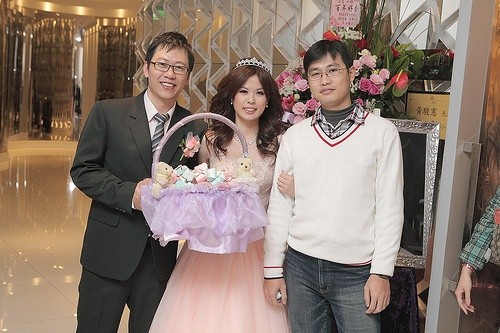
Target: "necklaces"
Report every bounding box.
[233,129,259,150]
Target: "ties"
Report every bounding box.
[151,113,170,161]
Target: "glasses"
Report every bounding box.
[305,67,347,81]
[149,61,191,75]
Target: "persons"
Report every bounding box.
[454,184,500,316]
[261,39,405,333]
[145,58,295,333]
[68,31,209,333]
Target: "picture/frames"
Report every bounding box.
[386,117,440,267]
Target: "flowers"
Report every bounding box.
[179,132,201,161]
[275,0,424,125]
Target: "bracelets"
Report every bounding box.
[461,262,476,272]
[375,274,390,279]
